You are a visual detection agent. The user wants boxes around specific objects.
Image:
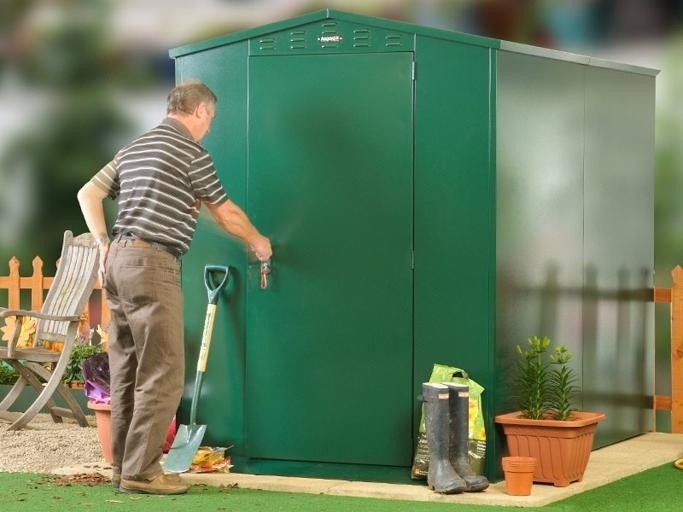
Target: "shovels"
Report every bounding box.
[162,264,231,473]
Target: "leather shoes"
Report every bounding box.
[113,473,191,495]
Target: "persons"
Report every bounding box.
[72,76,276,495]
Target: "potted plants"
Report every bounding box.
[493,335,606,487]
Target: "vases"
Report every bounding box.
[86,400,112,465]
[500,456,538,496]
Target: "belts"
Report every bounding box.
[116,235,179,257]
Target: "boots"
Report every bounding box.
[423,383,489,494]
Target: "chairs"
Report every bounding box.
[0,230,100,433]
